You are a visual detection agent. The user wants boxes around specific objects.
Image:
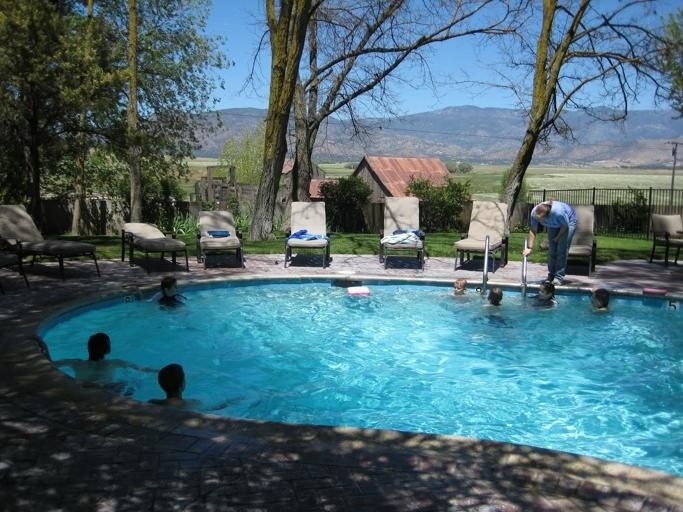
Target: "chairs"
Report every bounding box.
[566,203,597,277]
[650,213,683,267]
[379,196,425,270]
[284,201,333,269]
[196,210,246,270]
[453,200,509,273]
[121,222,191,277]
[1,203,101,289]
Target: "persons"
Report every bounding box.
[522,200,577,286]
[482,287,503,307]
[450,279,467,297]
[51,333,160,388]
[532,281,557,311]
[146,278,187,307]
[147,363,245,414]
[592,289,609,312]
